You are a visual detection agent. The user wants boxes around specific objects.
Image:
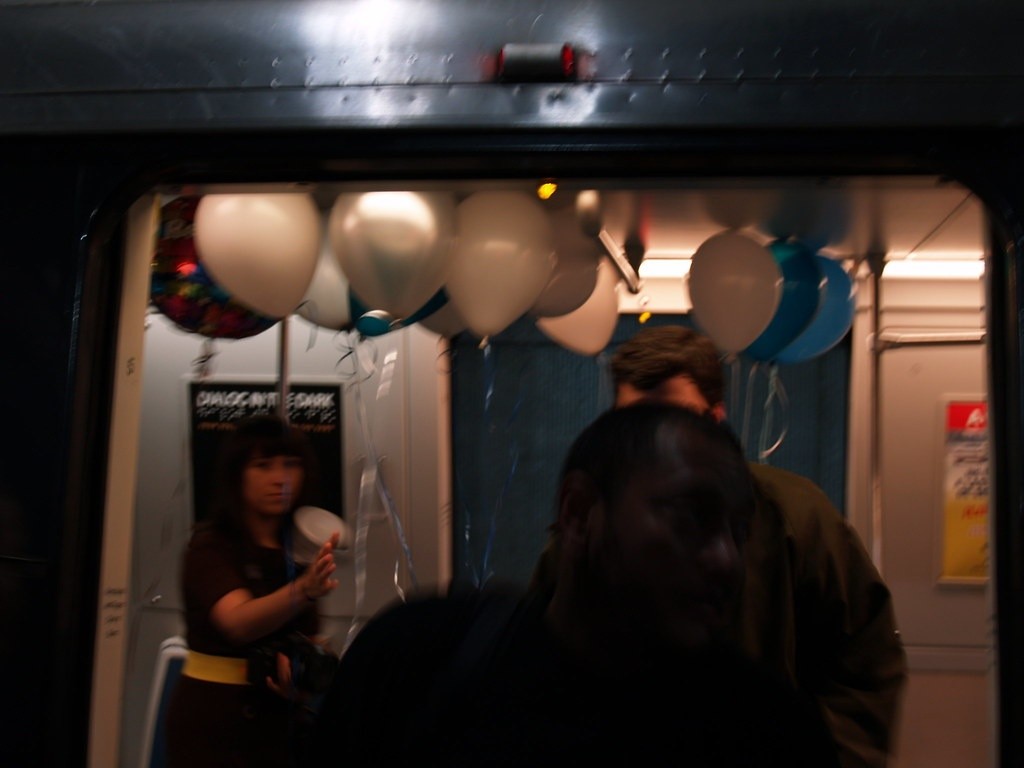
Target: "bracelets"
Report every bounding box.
[302,581,317,602]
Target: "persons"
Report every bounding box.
[152,325,906,768]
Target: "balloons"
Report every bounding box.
[690,234,853,367]
[150,188,619,356]
